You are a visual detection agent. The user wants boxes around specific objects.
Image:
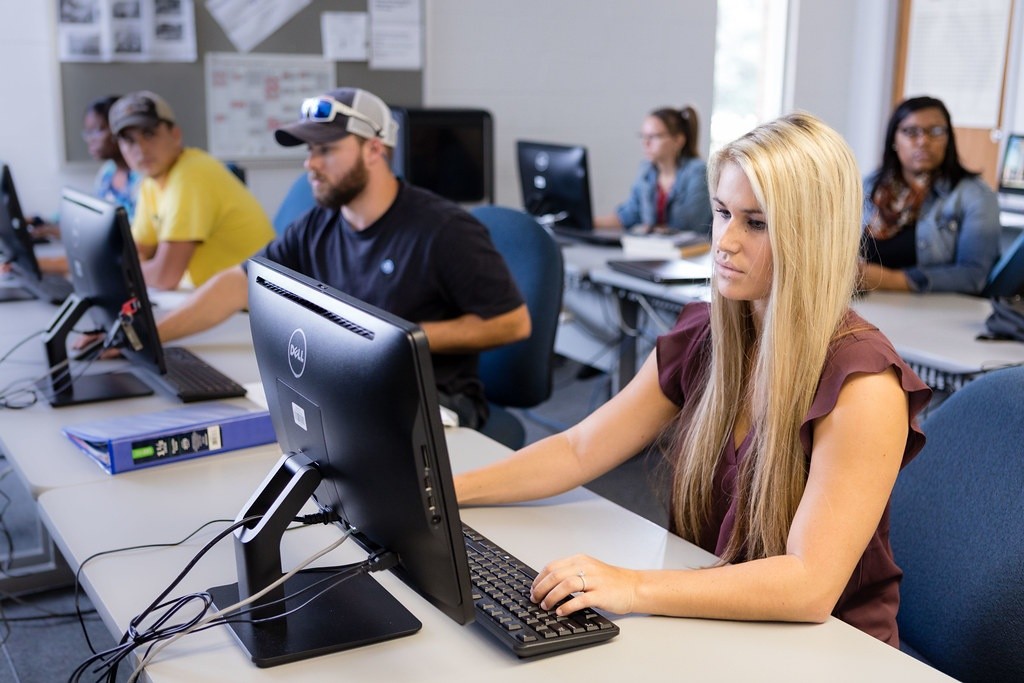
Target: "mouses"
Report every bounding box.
[69,339,103,361]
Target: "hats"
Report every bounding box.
[275,87,400,150]
[108,90,175,136]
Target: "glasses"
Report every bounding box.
[81,124,109,143]
[638,129,672,142]
[898,125,952,140]
[298,97,385,138]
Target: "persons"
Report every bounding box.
[25,97,141,241]
[0,89,276,313]
[592,106,714,237]
[858,95,1002,299]
[453,112,933,650]
[71,86,531,430]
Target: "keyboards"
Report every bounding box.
[461,520,622,658]
[20,272,74,305]
[121,349,249,403]
[551,225,621,246]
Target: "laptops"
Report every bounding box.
[607,259,713,284]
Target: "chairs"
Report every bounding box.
[272,170,318,237]
[890,366,1024,683]
[462,206,564,451]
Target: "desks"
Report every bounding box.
[545,228,1024,463]
[1,229,967,683]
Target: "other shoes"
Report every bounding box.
[578,363,606,380]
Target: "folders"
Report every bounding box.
[61,396,276,475]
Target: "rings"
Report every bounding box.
[577,570,585,592]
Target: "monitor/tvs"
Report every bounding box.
[994,128,1024,214]
[204,257,476,668]
[516,141,595,231]
[0,164,44,302]
[400,108,494,209]
[34,188,167,406]
